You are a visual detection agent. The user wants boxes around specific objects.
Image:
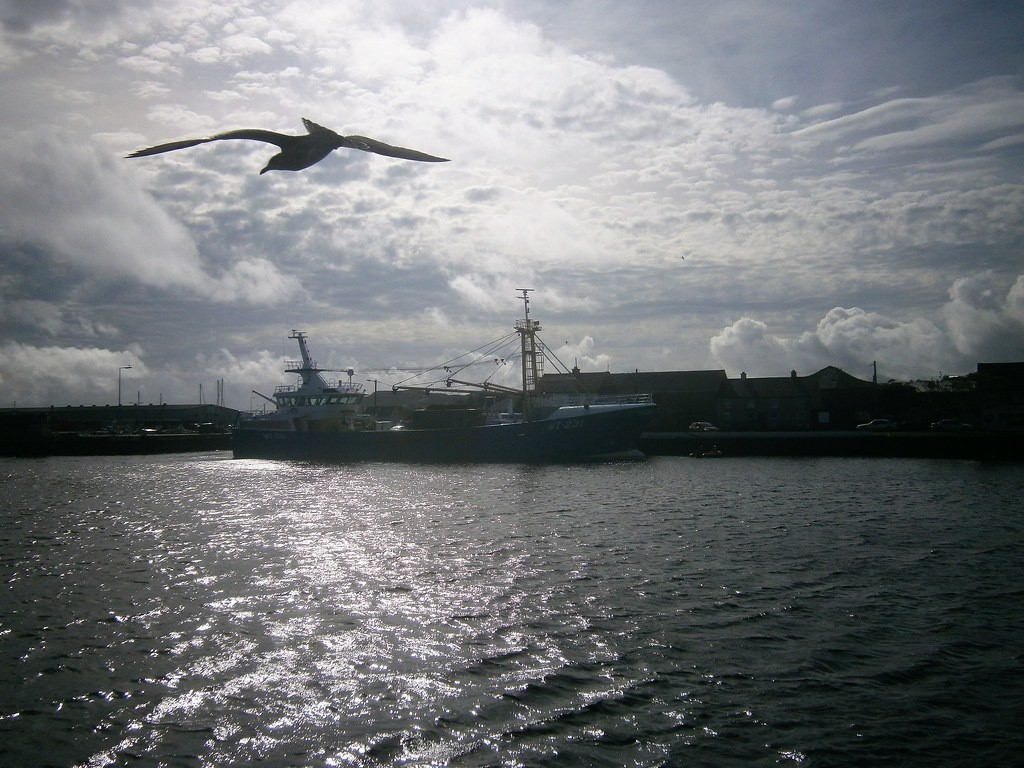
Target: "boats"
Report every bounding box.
[230,289,657,460]
[688,445,723,458]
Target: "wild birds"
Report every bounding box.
[123,116,453,175]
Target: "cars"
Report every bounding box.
[856,419,902,432]
[688,421,720,432]
[929,419,972,431]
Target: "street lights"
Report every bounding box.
[119,364,132,405]
